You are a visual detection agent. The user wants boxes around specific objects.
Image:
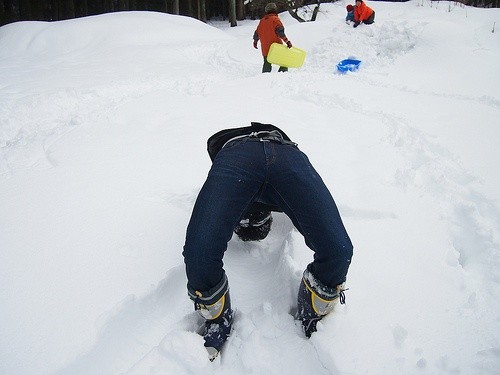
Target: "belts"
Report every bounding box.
[249,137,295,145]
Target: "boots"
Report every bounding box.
[300,268,348,338]
[187,269,232,359]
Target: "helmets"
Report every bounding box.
[234,210,272,241]
[265,3,276,13]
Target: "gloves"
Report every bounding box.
[287,41,292,48]
[254,40,258,49]
[353,21,360,27]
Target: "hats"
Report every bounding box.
[347,5,353,12]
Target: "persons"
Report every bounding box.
[182,122,353,363]
[253,3,293,73]
[353,0,375,28]
[345,4,356,25]
